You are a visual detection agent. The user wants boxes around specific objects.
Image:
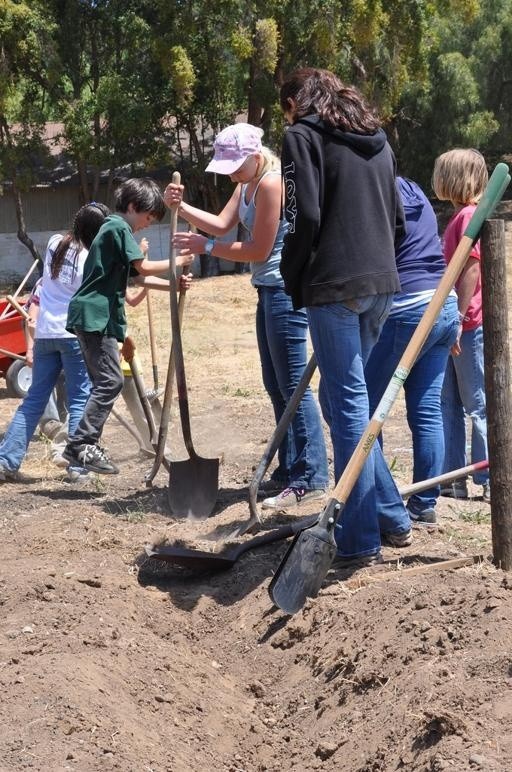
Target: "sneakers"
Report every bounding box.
[146,388,165,403]
[42,419,120,481]
[257,479,289,495]
[263,486,327,509]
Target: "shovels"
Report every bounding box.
[168,170,220,521]
[128,358,171,474]
[111,406,156,457]
[143,460,489,569]
[225,352,318,539]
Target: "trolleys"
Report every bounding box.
[0,295,34,399]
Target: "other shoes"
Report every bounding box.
[327,550,384,574]
[0,468,32,483]
[438,476,468,498]
[379,528,413,546]
[405,498,436,524]
[482,478,491,501]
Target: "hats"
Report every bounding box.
[204,123,263,175]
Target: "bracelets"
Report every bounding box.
[205,236,216,255]
[455,310,469,322]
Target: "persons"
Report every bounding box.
[163,119,328,511]
[62,177,192,476]
[0,202,111,483]
[430,147,504,504]
[318,172,462,529]
[279,60,415,569]
